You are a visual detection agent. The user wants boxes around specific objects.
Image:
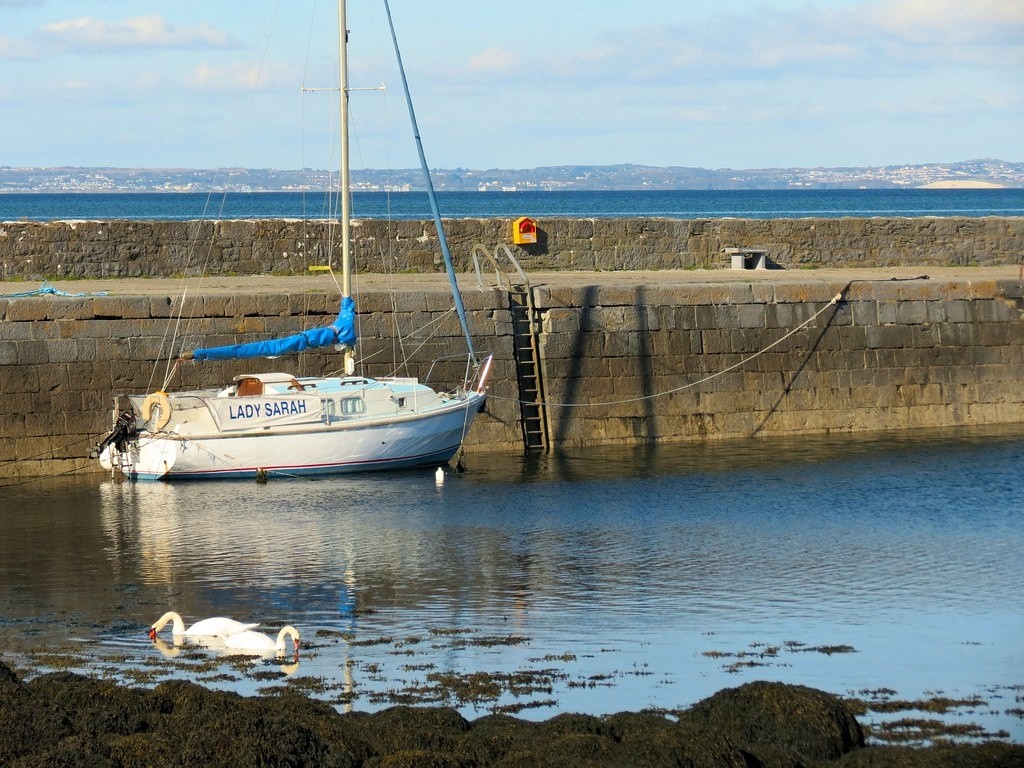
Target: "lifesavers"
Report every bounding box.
[141,391,173,431]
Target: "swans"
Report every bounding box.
[221,626,301,651]
[146,611,261,642]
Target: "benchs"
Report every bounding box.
[724,248,769,269]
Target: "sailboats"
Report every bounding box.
[96,1,495,481]
